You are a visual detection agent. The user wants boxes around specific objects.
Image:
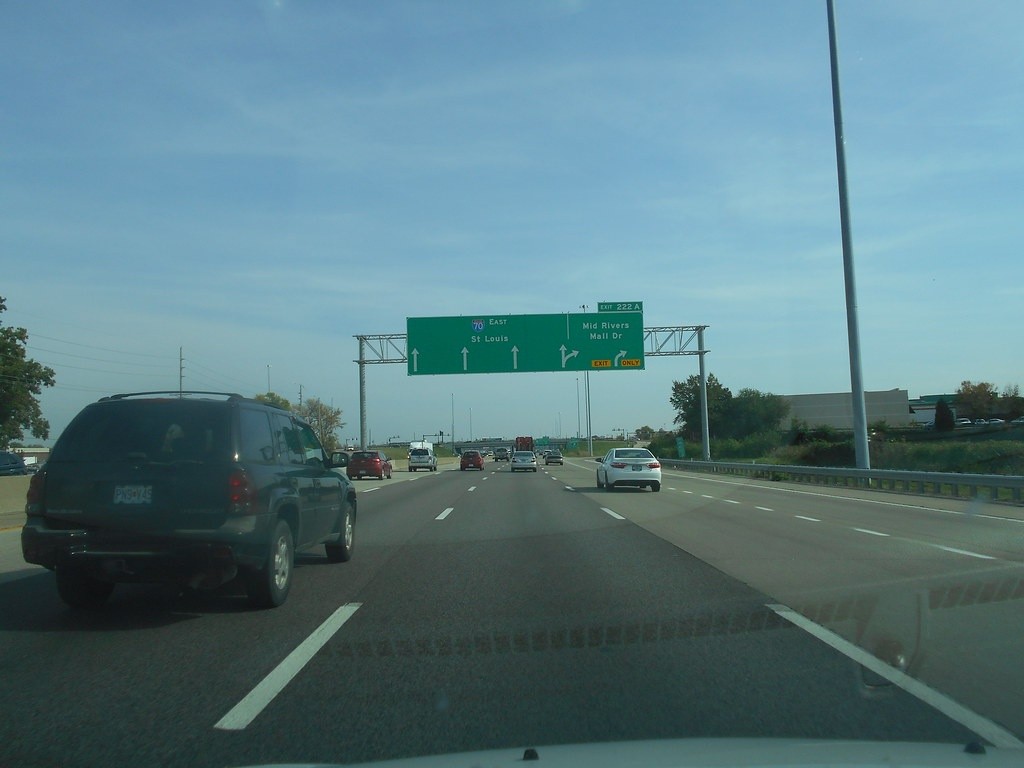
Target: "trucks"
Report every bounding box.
[515,437,534,452]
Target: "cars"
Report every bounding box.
[464,441,471,443]
[1011,416,1024,423]
[346,450,393,480]
[460,450,484,471]
[953,418,971,425]
[987,418,1006,424]
[488,451,493,456]
[25,463,43,473]
[510,451,537,472]
[907,423,926,428]
[539,451,543,455]
[974,419,989,424]
[507,450,511,454]
[545,450,563,465]
[595,448,661,492]
[922,421,935,426]
[346,444,354,451]
[637,437,641,441]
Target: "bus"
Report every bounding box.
[481,437,502,442]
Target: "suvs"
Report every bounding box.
[494,447,510,462]
[408,447,438,472]
[582,435,598,439]
[543,450,552,459]
[21,391,358,609]
[0,451,28,476]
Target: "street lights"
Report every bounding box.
[451,393,455,453]
[265,364,272,392]
[469,407,474,443]
[575,377,581,439]
